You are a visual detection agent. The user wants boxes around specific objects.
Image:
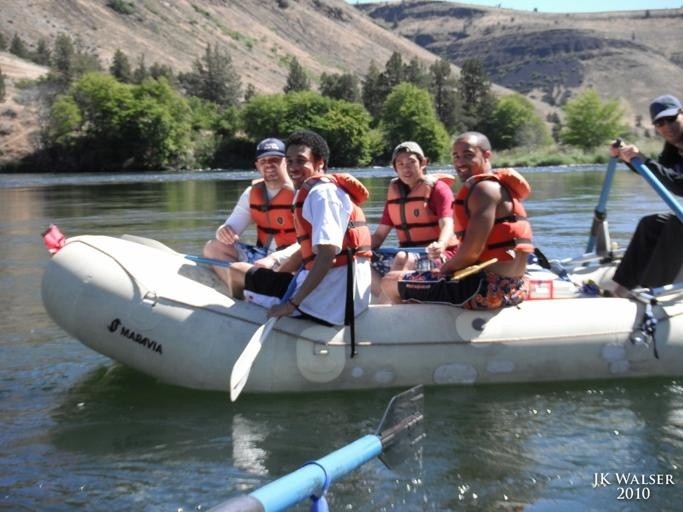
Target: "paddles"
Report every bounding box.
[586,138,621,255]
[193,384,425,511]
[121,233,230,267]
[374,245,428,255]
[453,258,499,280]
[229,257,307,404]
[627,157,683,225]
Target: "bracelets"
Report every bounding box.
[285,297,301,311]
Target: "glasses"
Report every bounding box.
[653,116,677,127]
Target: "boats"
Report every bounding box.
[40,235,683,395]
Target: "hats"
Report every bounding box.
[257,138,286,159]
[650,95,683,123]
[392,141,426,172]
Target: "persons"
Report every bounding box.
[204,138,303,288]
[372,141,458,305]
[609,95,683,297]
[228,129,373,328]
[378,131,534,311]
